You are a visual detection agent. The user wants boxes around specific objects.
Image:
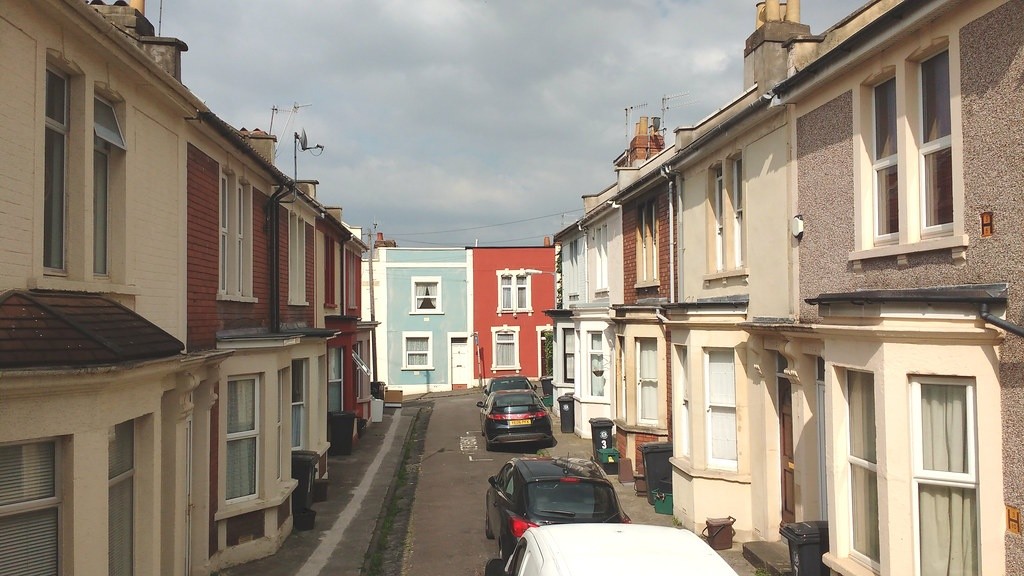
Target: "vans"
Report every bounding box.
[484,522,740,576]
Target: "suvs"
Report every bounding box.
[486,454,632,564]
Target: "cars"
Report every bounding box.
[476,389,553,451]
[483,377,537,401]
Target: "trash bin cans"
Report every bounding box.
[293,507,316,530]
[637,442,673,505]
[702,516,736,550]
[327,411,355,456]
[588,417,614,460]
[651,477,673,514]
[543,395,553,407]
[633,475,647,497]
[314,478,329,502]
[370,381,386,408]
[596,448,621,475]
[779,520,829,576]
[291,450,320,514]
[557,395,574,433]
[540,376,553,395]
